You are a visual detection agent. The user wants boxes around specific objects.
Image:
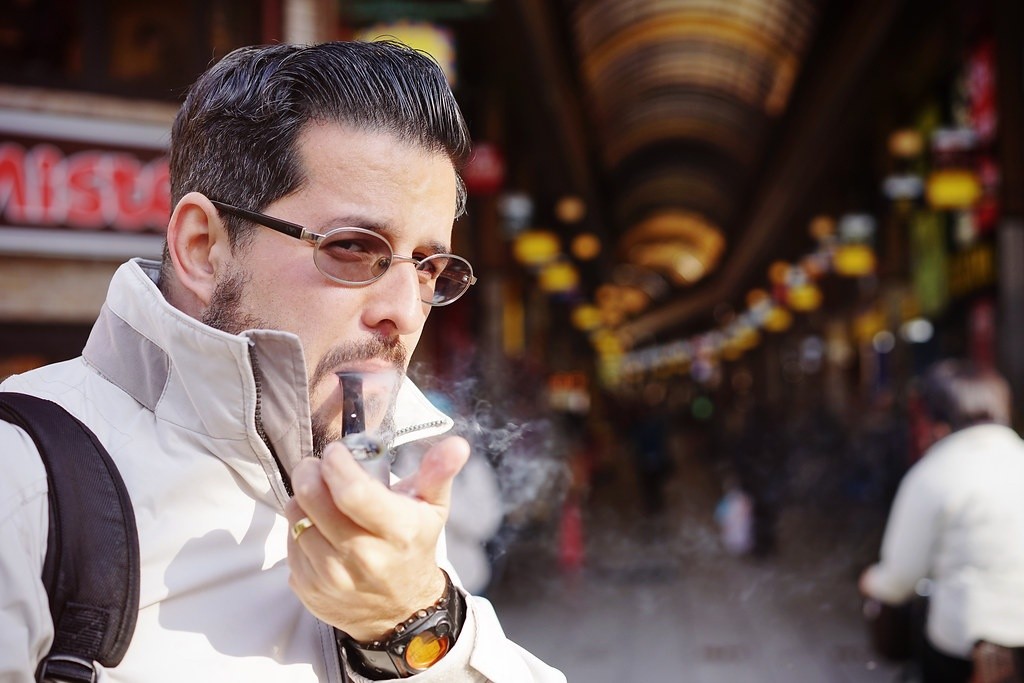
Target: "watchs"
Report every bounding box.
[339,568,466,682]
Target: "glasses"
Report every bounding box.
[208,200,479,307]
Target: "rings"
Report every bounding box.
[291,518,315,540]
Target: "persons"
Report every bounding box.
[0,38,570,683]
[857,359,1024,683]
